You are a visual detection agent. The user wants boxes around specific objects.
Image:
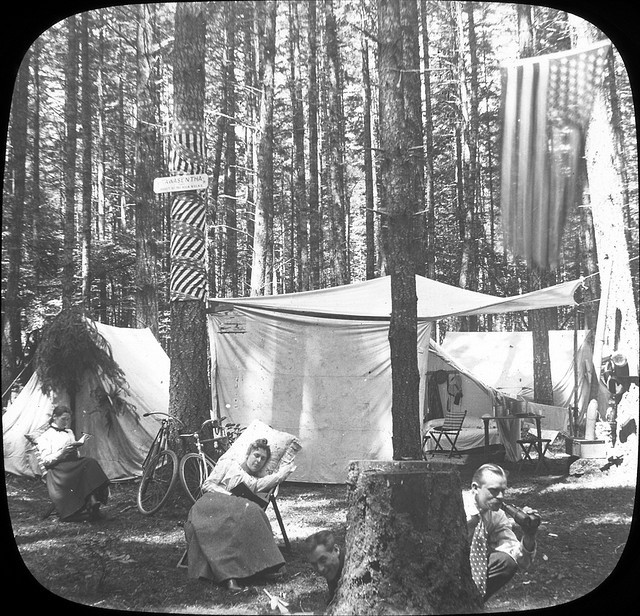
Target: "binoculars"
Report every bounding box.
[501,500,541,529]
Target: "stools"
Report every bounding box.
[421,436,430,461]
[517,440,550,474]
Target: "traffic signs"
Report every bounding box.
[153,174,208,194]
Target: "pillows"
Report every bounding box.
[218,420,295,479]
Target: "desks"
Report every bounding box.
[480,414,545,463]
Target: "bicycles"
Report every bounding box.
[136,411,192,518]
[179,416,248,505]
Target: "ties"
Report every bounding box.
[469,512,490,597]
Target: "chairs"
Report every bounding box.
[429,409,467,459]
[25,431,105,520]
[177,486,291,569]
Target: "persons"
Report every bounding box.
[303,530,346,599]
[457,462,542,603]
[32,405,111,521]
[179,439,297,591]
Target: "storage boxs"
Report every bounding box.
[573,440,605,459]
